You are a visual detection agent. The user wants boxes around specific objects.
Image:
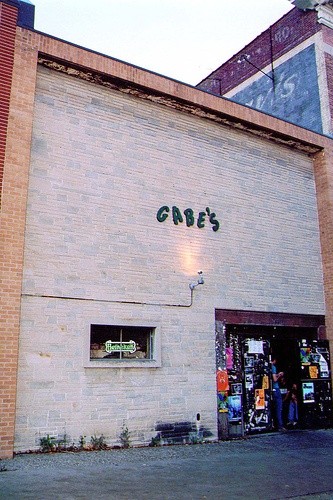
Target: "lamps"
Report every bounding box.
[189,269,205,290]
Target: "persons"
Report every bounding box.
[269,356,287,432]
[287,382,299,426]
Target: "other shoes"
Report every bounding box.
[278,427,288,432]
[287,421,298,426]
